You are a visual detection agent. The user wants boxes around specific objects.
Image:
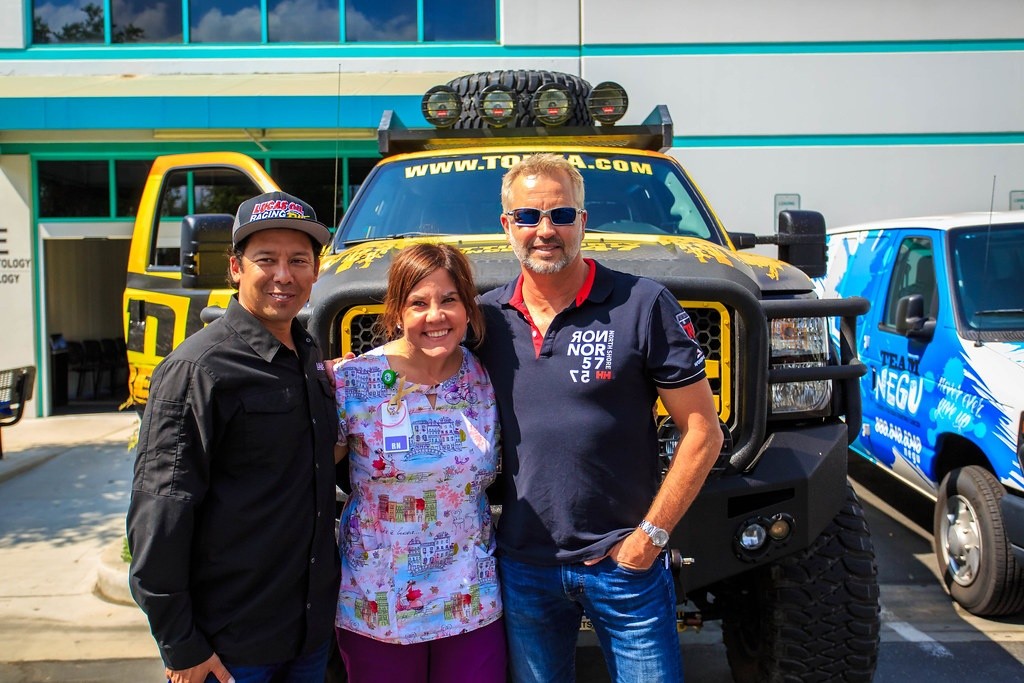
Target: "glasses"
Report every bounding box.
[504,206,584,227]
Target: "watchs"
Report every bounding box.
[638,519,670,548]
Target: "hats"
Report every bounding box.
[232,191,331,250]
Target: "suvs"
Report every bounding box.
[121,66,885,683]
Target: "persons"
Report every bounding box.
[125,192,352,683]
[333,243,508,683]
[323,153,725,683]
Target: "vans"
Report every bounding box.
[813,209,1024,621]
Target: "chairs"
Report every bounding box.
[585,201,630,230]
[66,336,128,400]
[898,255,936,319]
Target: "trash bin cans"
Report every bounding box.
[51,333,129,408]
